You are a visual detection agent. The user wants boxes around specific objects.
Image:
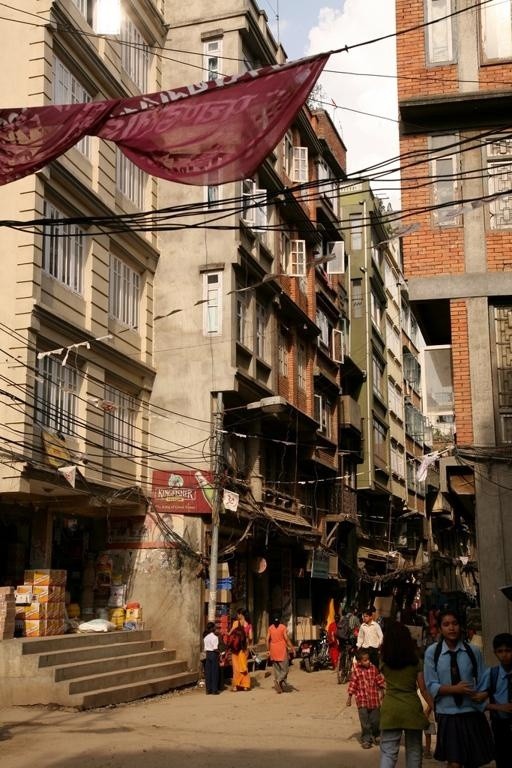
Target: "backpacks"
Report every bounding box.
[227,621,247,654]
[334,613,354,640]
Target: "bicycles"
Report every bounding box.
[332,638,355,686]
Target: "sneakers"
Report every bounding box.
[274,680,284,694]
[363,739,371,748]
[374,737,382,744]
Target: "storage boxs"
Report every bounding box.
[295,614,320,641]
[1,563,71,641]
[200,556,236,645]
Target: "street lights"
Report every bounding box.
[208,393,289,631]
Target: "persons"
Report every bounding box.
[224,607,254,692]
[423,610,489,768]
[161,514,182,543]
[203,622,221,695]
[346,652,386,749]
[266,615,296,693]
[471,633,512,768]
[378,621,433,768]
[327,603,385,684]
[416,651,436,759]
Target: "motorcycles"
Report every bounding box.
[299,621,334,674]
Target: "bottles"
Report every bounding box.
[194,471,225,514]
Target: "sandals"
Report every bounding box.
[424,751,433,760]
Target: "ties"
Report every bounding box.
[505,676,512,704]
[449,651,465,708]
